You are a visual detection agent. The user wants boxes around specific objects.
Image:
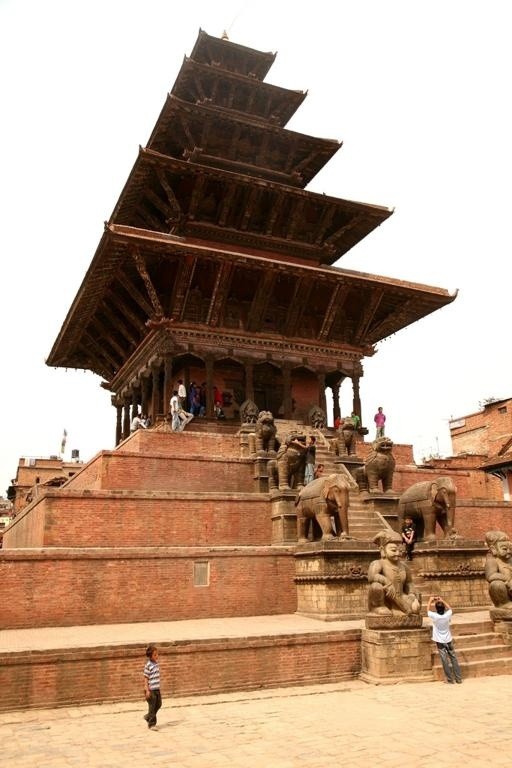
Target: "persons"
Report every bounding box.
[314,464,324,479]
[400,515,417,561]
[427,596,461,684]
[143,647,162,731]
[375,407,386,438]
[351,411,360,430]
[368,530,421,615]
[335,416,342,429]
[291,436,315,485]
[130,380,226,432]
[485,531,512,607]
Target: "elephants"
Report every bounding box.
[399,476,458,540]
[294,475,350,544]
